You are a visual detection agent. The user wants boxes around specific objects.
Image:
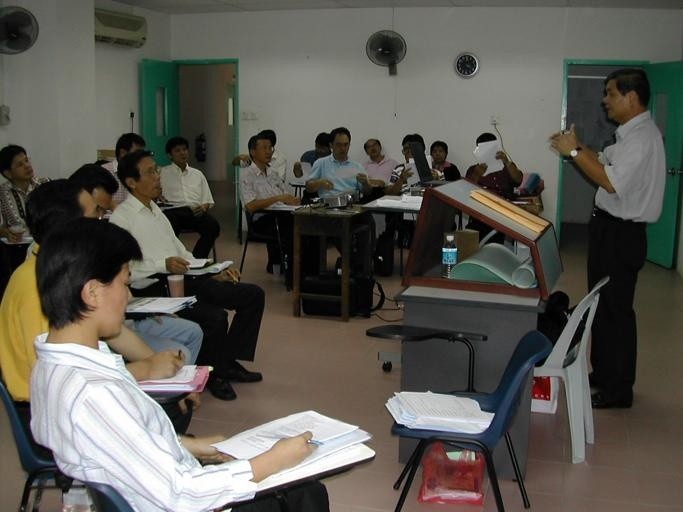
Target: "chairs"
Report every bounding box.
[531,275,612,467]
[237,153,414,322]
[87,482,134,512]
[0,380,56,512]
[389,331,552,512]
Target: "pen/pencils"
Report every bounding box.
[178,351,182,361]
[256,431,324,445]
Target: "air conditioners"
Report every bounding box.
[93,7,147,48]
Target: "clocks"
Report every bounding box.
[454,53,479,78]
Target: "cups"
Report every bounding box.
[9,225,22,242]
[166,274,184,297]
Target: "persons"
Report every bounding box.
[231,126,461,291]
[464,133,522,244]
[63,164,206,440]
[100,133,221,259]
[27,217,329,510]
[0,145,55,292]
[105,149,263,400]
[0,176,203,466]
[545,65,668,411]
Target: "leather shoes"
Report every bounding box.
[230,362,262,382]
[207,377,236,400]
[591,393,632,409]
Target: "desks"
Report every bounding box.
[135,353,210,407]
[363,319,488,356]
[160,254,231,282]
[217,419,373,491]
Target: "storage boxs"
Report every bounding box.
[531,377,560,417]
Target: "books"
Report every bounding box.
[207,410,375,477]
[123,294,197,315]
[118,361,213,393]
[468,181,549,234]
[384,388,497,431]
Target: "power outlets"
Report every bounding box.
[128,108,136,120]
[489,114,498,125]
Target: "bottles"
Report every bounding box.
[460,446,472,461]
[441,234,457,276]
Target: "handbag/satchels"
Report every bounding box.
[302,272,375,318]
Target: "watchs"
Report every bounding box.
[568,146,581,161]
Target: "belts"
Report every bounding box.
[591,208,623,223]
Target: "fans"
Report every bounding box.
[0,6,38,59]
[364,30,407,77]
